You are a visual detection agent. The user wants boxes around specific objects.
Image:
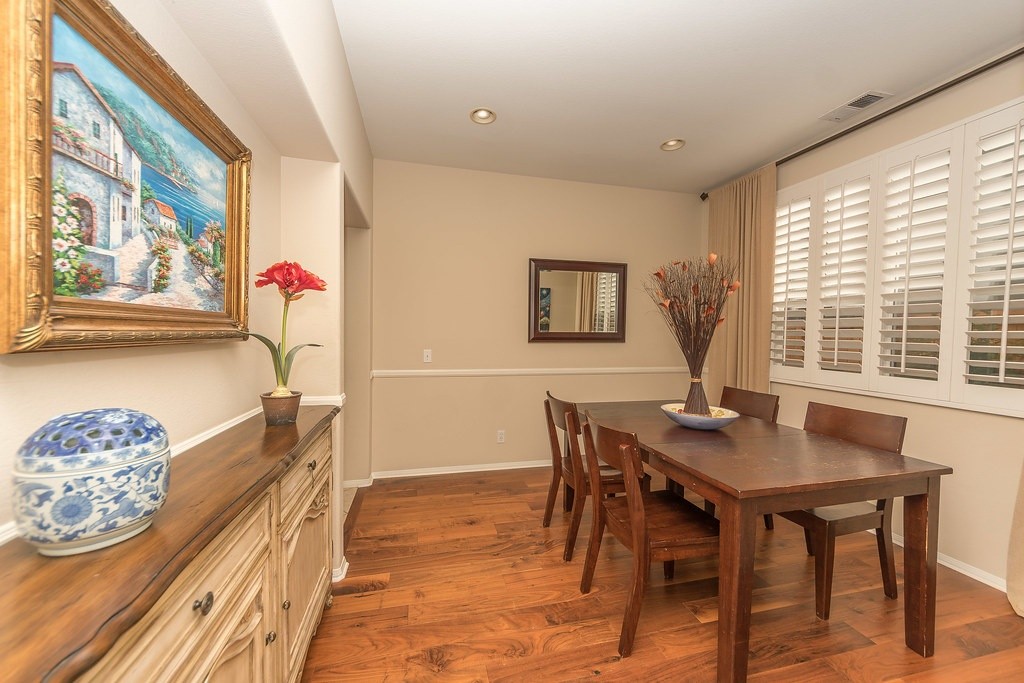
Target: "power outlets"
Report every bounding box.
[424,349,433,363]
[497,431,505,444]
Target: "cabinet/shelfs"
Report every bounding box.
[1,404,340,683]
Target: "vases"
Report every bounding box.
[260,391,302,426]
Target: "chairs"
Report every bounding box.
[803,401,908,620]
[542,390,614,528]
[580,410,721,658]
[704,386,780,530]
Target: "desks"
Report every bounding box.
[571,398,953,683]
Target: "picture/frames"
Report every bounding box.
[0,0,253,355]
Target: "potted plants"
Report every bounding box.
[640,253,741,429]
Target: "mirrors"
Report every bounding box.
[528,258,628,344]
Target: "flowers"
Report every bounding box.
[233,259,327,396]
